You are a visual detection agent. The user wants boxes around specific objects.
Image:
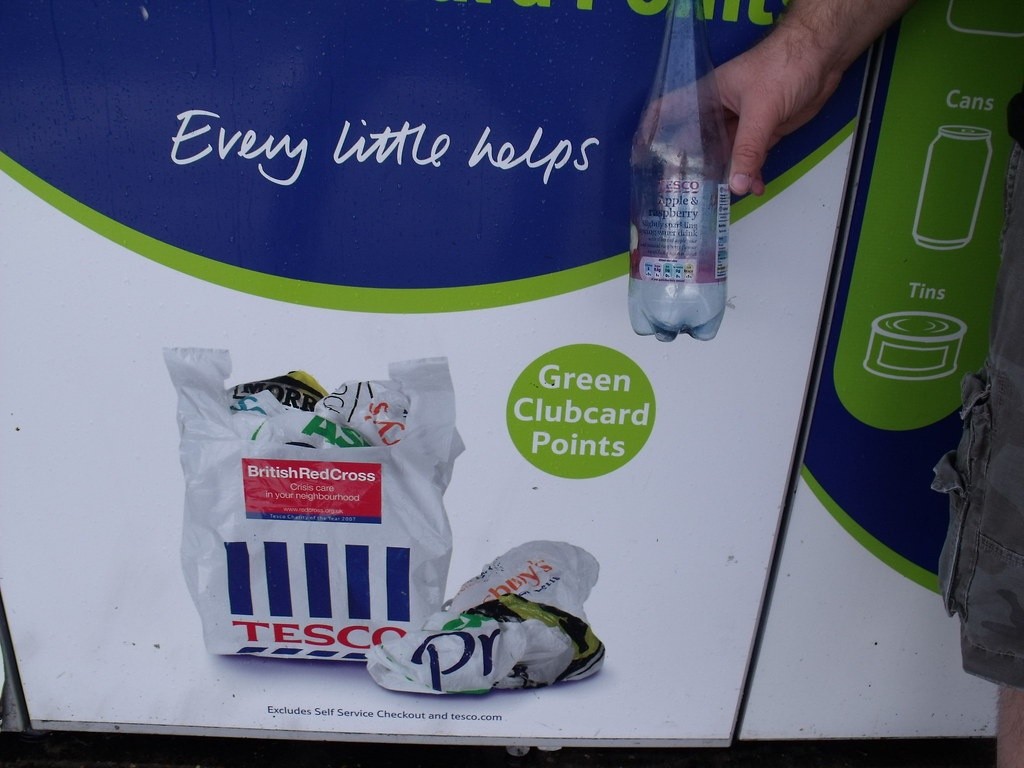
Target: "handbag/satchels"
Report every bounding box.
[163,346,467,661]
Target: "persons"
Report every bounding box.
[636,0,1024,768]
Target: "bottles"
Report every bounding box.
[628,0,732,342]
